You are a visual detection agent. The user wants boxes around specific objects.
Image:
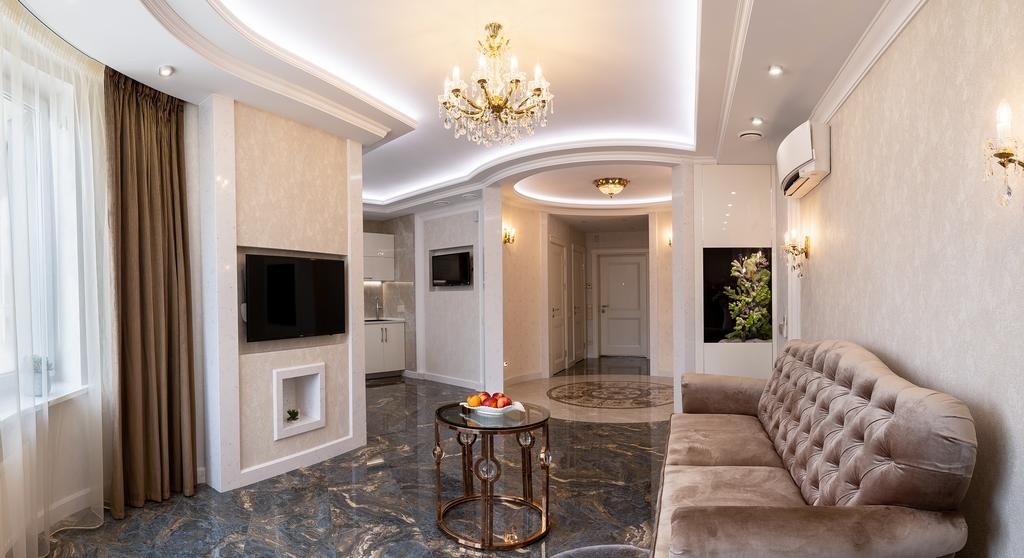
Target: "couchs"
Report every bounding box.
[650,339,977,558]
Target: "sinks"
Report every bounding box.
[365,319,391,322]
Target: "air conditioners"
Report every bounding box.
[775,120,832,200]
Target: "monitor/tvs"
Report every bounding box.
[244,254,346,342]
[432,252,470,286]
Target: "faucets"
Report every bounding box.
[376,299,382,320]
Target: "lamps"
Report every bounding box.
[502,227,515,244]
[593,178,630,198]
[782,232,809,279]
[438,23,554,149]
[983,99,1024,208]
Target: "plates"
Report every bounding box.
[460,412,524,427]
[459,400,526,416]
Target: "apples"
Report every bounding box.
[467,392,512,409]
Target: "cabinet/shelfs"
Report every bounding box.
[364,322,405,379]
[362,233,395,282]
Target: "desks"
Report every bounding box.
[434,402,551,550]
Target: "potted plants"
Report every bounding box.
[33,355,54,398]
